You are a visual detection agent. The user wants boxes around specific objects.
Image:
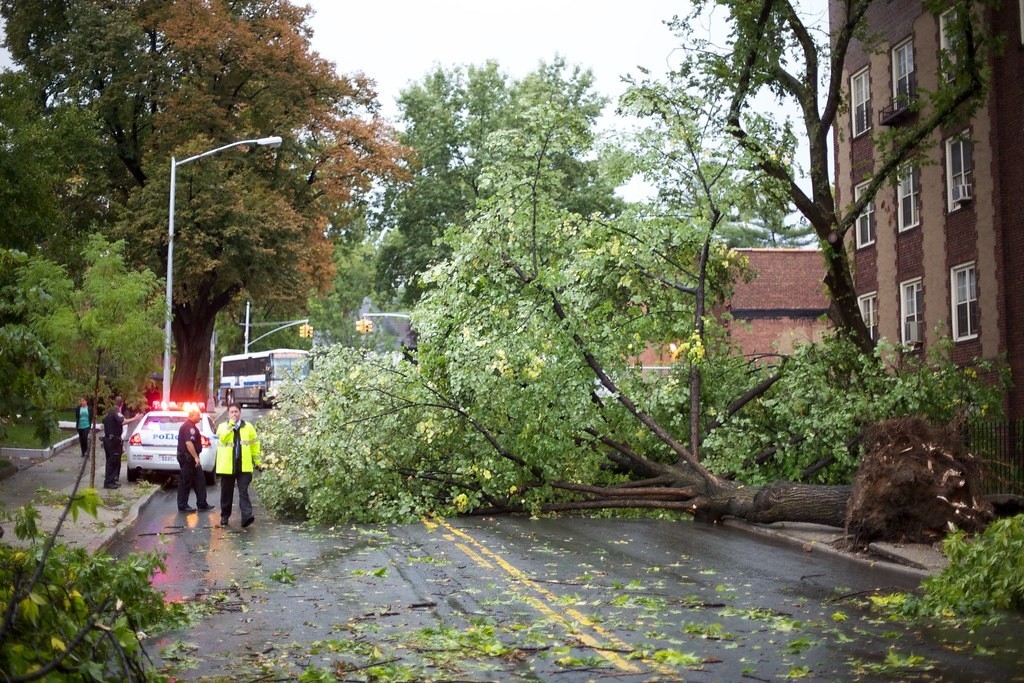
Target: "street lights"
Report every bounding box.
[162,133,283,399]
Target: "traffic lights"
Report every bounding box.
[355,320,365,335]
[306,324,313,338]
[299,324,307,338]
[365,319,373,334]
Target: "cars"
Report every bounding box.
[127,400,218,486]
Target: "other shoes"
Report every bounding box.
[198,504,215,511]
[180,506,196,512]
[241,514,255,528]
[221,516,228,524]
[104,482,122,489]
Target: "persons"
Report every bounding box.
[176,409,216,513]
[214,401,264,529]
[75,396,94,458]
[101,395,144,490]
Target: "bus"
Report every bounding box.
[217,347,313,409]
